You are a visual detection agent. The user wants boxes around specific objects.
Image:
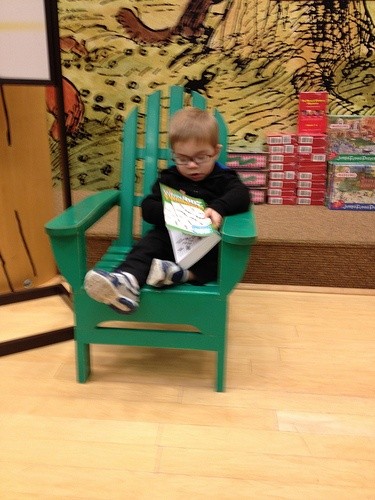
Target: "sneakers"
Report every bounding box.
[84,268,140,316]
[146,257,184,287]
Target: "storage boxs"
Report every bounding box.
[229,91,375,211]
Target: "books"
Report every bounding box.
[159,182,222,270]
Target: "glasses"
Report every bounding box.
[171,149,217,166]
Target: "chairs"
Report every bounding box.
[44,84,259,392]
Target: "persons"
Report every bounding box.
[83,106,251,315]
